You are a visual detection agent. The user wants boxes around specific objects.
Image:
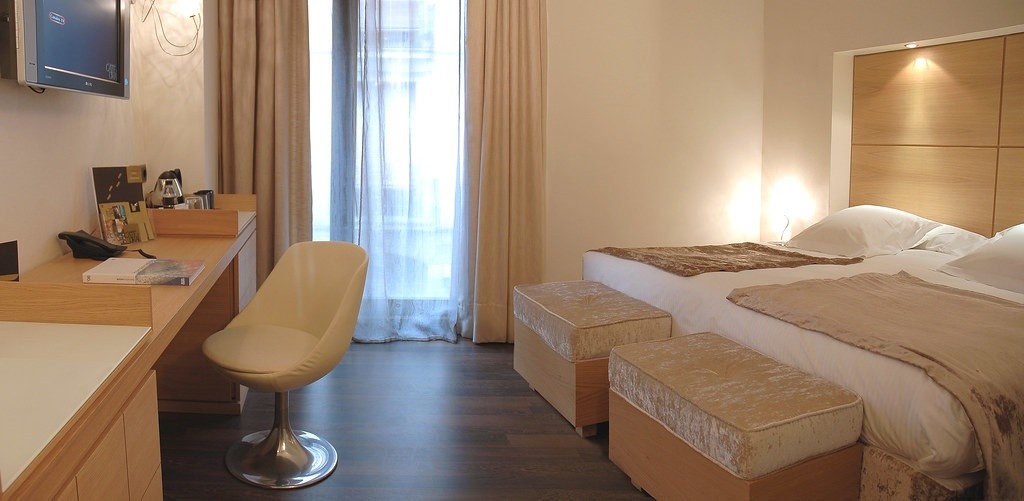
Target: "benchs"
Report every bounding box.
[513,280,671,438]
[608,331,865,501]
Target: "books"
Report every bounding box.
[82,257,206,286]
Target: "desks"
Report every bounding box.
[0,193,259,501]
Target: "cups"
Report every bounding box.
[185,196,204,209]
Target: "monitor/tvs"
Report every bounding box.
[15,0,131,100]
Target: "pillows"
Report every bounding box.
[936,223,1024,293]
[786,203,956,259]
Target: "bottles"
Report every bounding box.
[162,181,177,209]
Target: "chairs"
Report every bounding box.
[203,241,369,489]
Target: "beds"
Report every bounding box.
[581,225,1024,501]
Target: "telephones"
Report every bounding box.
[56,229,127,261]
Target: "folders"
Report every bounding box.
[82,257,206,286]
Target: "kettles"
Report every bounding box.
[153,169,183,205]
